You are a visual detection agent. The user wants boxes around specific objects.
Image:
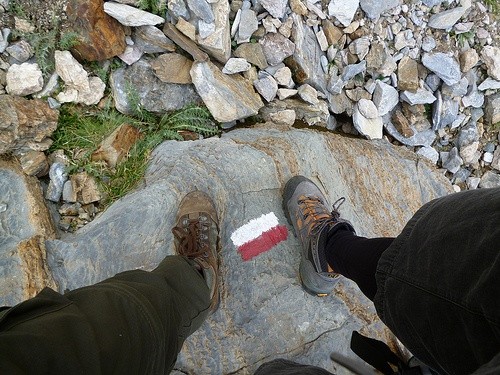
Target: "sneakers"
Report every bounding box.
[282,175,356,298]
[173,192,220,317]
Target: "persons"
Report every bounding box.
[1,175,500,375]
